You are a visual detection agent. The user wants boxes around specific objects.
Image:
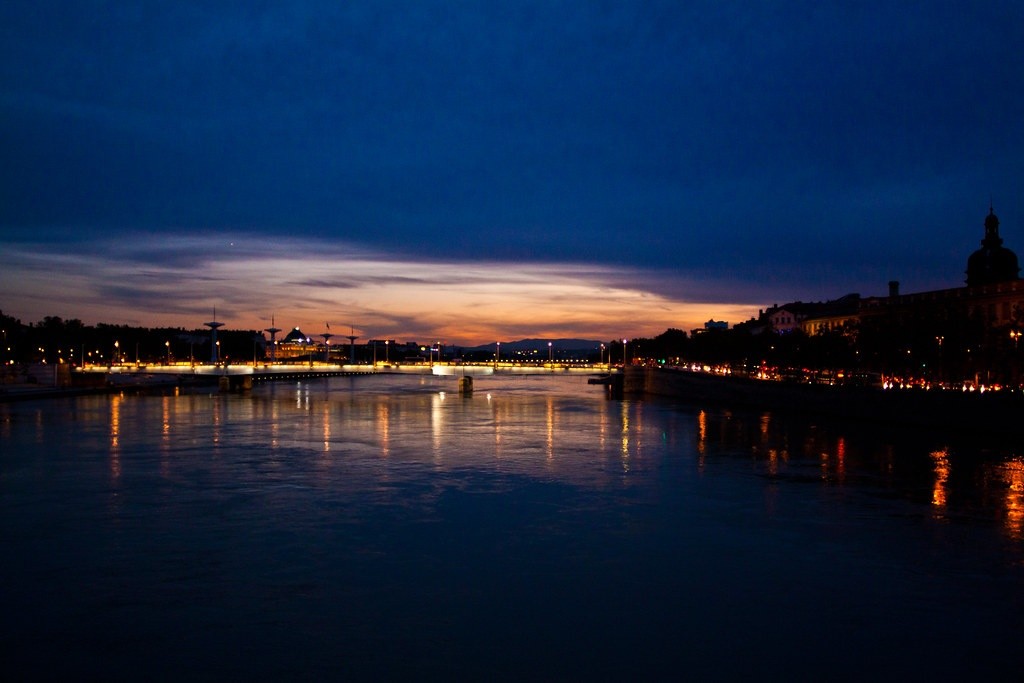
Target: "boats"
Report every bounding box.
[459,376,474,391]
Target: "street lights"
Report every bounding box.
[165,341,170,366]
[385,340,389,363]
[216,341,220,364]
[548,343,551,360]
[623,340,627,363]
[497,342,500,361]
[115,341,118,370]
[275,341,278,364]
